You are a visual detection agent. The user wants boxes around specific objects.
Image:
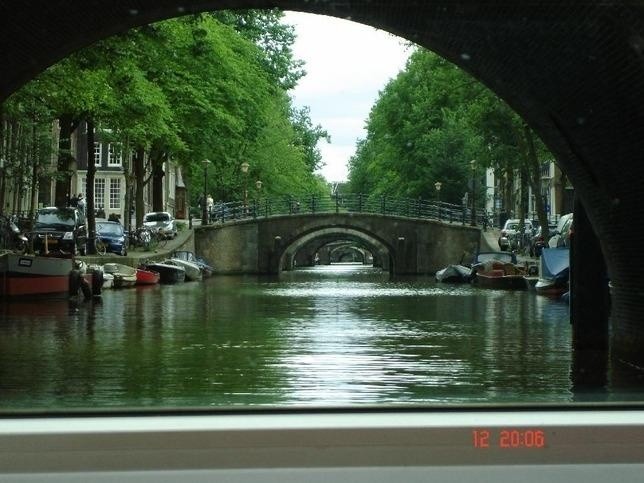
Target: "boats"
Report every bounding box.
[0,253,213,301]
[435,257,570,293]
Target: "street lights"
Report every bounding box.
[434,182,443,219]
[255,180,262,214]
[201,159,212,224]
[241,161,249,220]
[469,159,478,227]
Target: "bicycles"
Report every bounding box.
[130,229,168,251]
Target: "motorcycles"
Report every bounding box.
[0,215,29,250]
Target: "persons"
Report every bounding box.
[497,229,507,250]
[108,213,122,223]
[63,192,85,215]
[197,193,204,218]
[206,193,214,219]
[96,207,106,219]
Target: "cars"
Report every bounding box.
[498,212,575,252]
[32,206,129,255]
[142,211,178,239]
